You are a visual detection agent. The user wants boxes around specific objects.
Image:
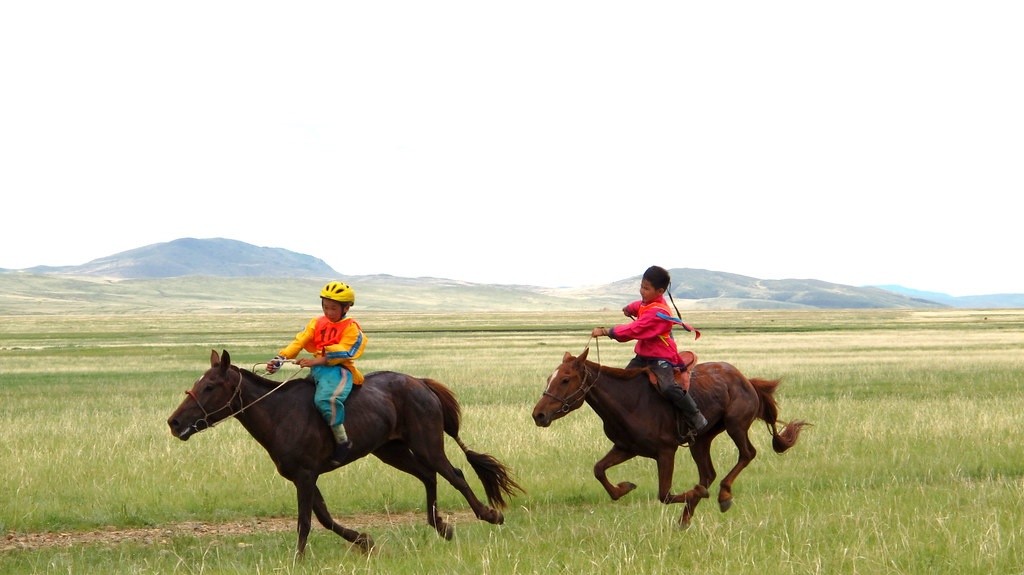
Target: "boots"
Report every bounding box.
[676,390,707,430]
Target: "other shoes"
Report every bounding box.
[332,441,352,465]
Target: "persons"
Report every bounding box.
[266,280,366,451]
[592,264,709,431]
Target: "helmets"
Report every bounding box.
[319,282,355,306]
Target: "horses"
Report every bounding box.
[532,348,815,531]
[168,349,526,561]
